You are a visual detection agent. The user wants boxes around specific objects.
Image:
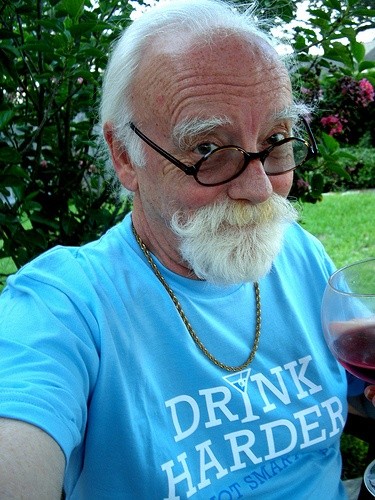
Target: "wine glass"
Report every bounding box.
[321,258,375,496]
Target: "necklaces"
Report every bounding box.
[131,224,261,372]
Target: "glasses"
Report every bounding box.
[129,114,319,187]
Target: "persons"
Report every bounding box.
[0,2,375,500]
